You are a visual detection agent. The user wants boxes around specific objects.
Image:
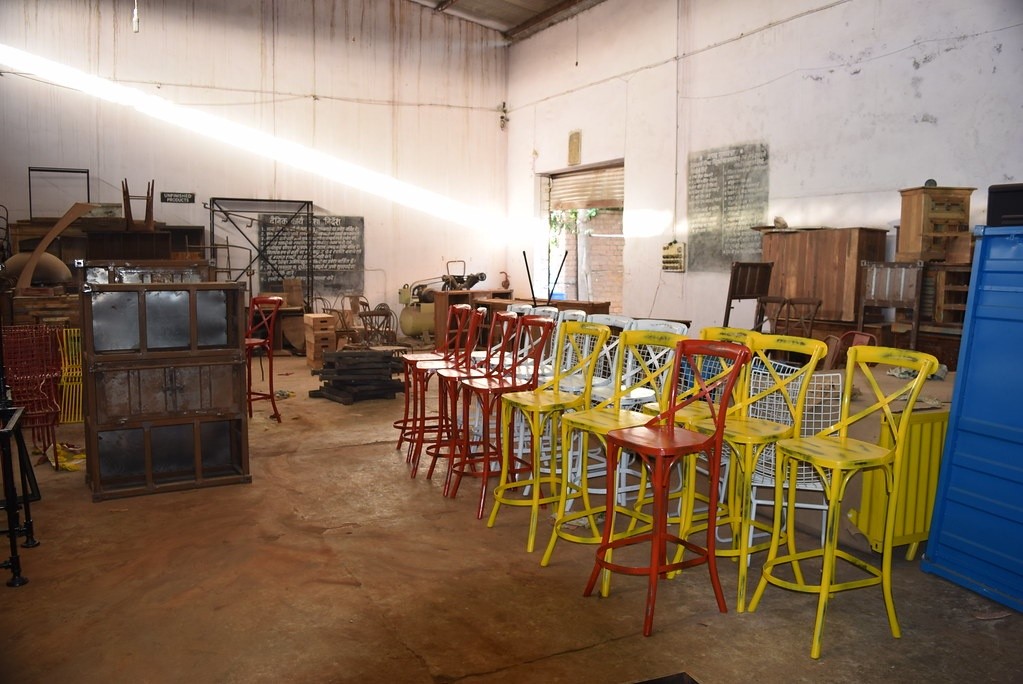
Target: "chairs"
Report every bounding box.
[449,313,559,501]
[482,321,613,553]
[662,329,828,598]
[456,301,534,456]
[570,317,687,508]
[783,296,822,338]
[403,310,487,479]
[743,344,941,658]
[672,356,805,548]
[489,304,561,480]
[243,294,284,429]
[753,294,789,333]
[2,322,85,463]
[634,326,762,525]
[582,338,752,634]
[393,302,472,454]
[426,309,521,497]
[536,329,685,594]
[562,312,633,500]
[521,306,589,497]
[316,292,414,357]
[734,370,848,569]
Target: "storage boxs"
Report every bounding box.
[302,312,337,371]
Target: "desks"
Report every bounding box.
[432,289,515,350]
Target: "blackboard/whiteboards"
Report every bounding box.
[257,214,366,297]
[685,141,768,274]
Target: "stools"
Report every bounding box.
[120,179,156,233]
